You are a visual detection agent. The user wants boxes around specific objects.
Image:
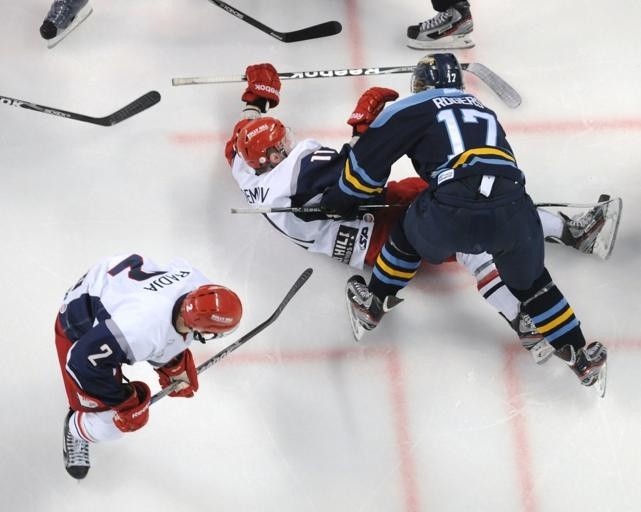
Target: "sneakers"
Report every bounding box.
[407,3,474,40]
[63,411,90,478]
[547,194,610,256]
[40,0,89,39]
[517,312,546,349]
[554,340,605,386]
[348,276,383,330]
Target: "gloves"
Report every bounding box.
[243,64,281,108]
[321,189,357,217]
[347,86,399,126]
[159,349,198,398]
[114,381,151,432]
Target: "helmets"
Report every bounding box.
[238,118,294,170]
[182,284,242,334]
[413,53,463,92]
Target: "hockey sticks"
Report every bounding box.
[0,90,161,126]
[149,268,313,404]
[172,62,522,109]
[231,203,611,213]
[211,0,343,43]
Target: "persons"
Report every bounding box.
[223,63,623,367]
[320,52,610,398]
[54,255,243,482]
